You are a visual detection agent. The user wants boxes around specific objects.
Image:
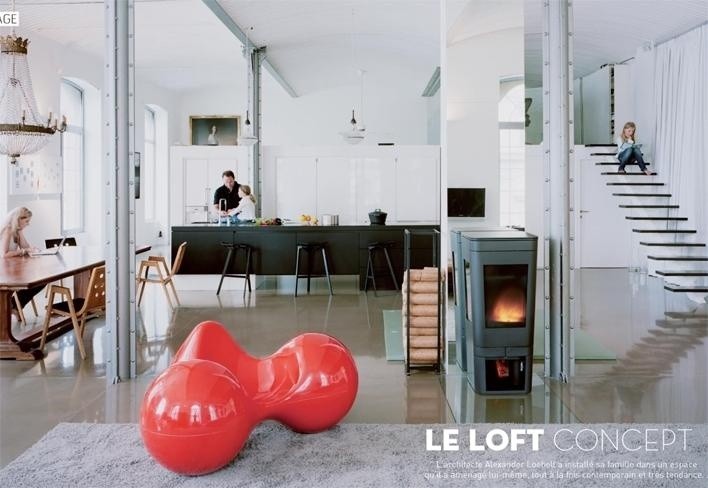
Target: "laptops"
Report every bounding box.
[32,235,66,255]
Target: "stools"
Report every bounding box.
[365,242,400,296]
[294,241,333,296]
[216,242,256,296]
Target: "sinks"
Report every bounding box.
[191,221,236,225]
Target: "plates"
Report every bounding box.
[284,220,297,223]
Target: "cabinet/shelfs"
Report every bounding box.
[169,147,441,228]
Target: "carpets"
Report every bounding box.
[0,423,708,488]
[381,308,404,362]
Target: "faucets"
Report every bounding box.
[218,199,227,225]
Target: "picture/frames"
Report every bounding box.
[134,151,140,199]
[189,115,240,146]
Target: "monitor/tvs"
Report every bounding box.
[135,152,140,199]
[448,188,485,221]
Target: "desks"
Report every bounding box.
[0,246,150,360]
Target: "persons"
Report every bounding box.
[227,186,255,223]
[213,171,242,222]
[616,122,652,175]
[0,206,47,321]
[208,123,220,145]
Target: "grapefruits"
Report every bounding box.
[299,214,311,221]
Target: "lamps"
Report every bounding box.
[0,0,67,166]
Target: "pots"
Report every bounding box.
[322,214,339,225]
[368,209,388,223]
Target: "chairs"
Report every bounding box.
[10,291,38,324]
[134,241,188,308]
[39,266,105,360]
[45,238,77,302]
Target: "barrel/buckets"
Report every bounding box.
[370,208,387,224]
